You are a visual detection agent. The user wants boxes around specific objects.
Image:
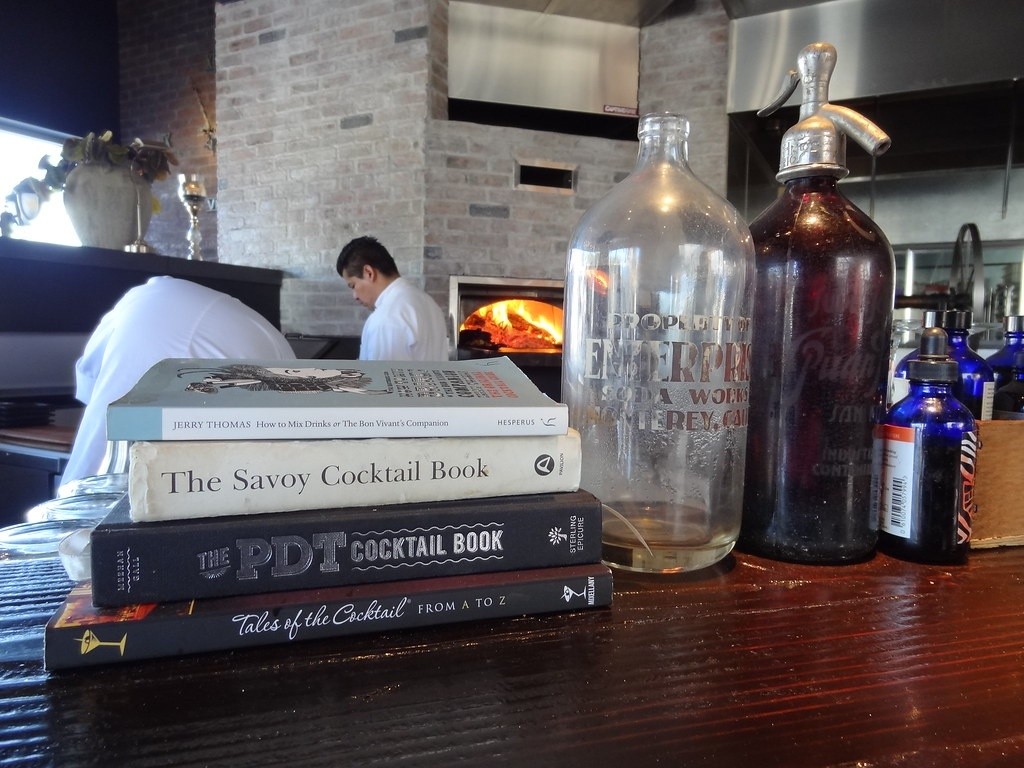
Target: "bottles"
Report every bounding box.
[738,44,894,565]
[875,326,980,564]
[985,315,1024,421]
[893,307,997,421]
[560,112,758,574]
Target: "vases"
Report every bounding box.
[65,158,155,253]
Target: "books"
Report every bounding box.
[44,356,614,671]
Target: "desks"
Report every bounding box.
[0,553,1024,768]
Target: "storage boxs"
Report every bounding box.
[968,407,1024,549]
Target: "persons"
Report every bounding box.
[337,236,448,362]
[58,275,296,486]
